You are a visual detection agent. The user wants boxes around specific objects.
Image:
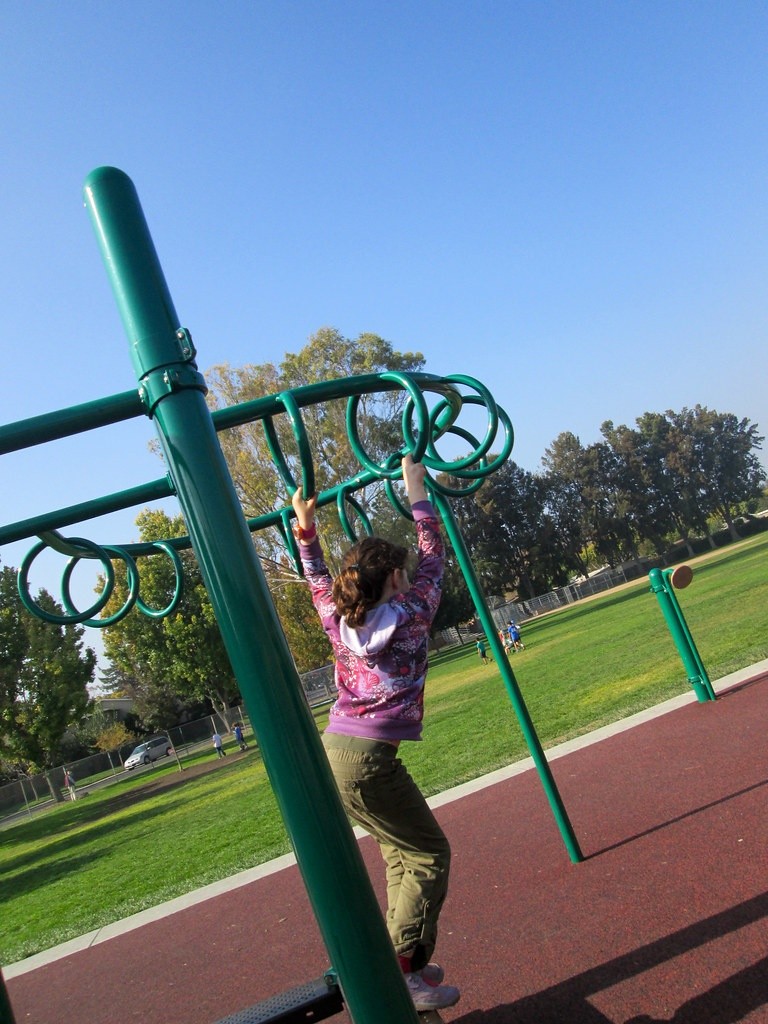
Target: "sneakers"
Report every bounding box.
[406,974,460,1011]
[413,963,444,986]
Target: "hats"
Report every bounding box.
[507,621,513,624]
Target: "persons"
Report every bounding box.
[64,770,78,802]
[503,619,526,655]
[290,452,461,1013]
[234,721,248,752]
[475,637,495,666]
[212,730,226,760]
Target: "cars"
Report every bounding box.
[124,736,172,771]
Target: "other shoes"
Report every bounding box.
[515,650,519,653]
[223,755,226,757]
[523,646,525,650]
[219,757,222,759]
